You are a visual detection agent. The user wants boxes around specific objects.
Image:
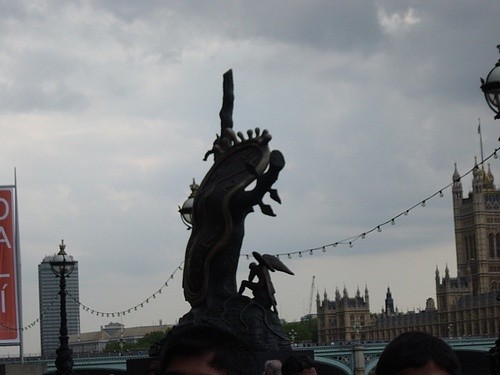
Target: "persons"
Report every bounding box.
[145,323,261,375]
[263,354,319,375]
[239,252,294,307]
[376,332,464,375]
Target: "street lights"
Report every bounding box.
[48,239,78,375]
[288,328,298,341]
